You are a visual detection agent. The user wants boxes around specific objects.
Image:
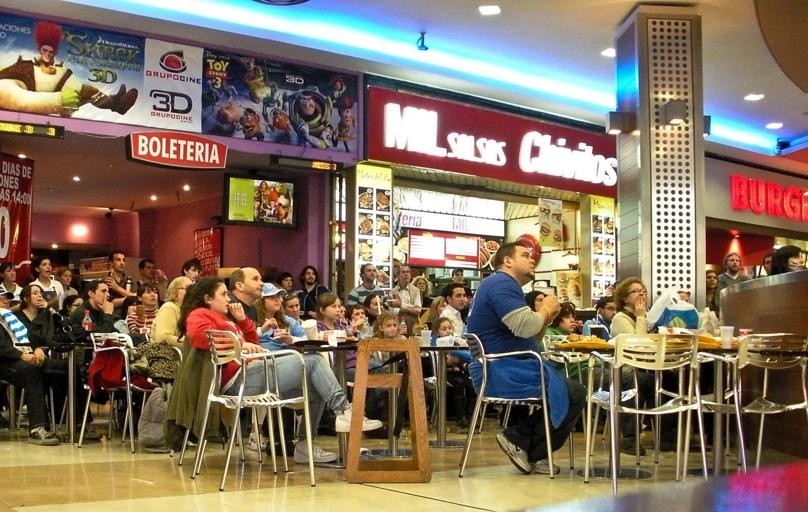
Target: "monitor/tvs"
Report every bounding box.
[222,172,298,229]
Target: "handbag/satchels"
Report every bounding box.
[645,287,702,333]
[27,308,87,352]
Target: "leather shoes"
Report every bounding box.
[455,412,479,429]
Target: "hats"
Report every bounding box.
[261,282,287,299]
[452,267,463,274]
[0,287,14,299]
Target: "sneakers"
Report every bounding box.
[76,422,103,439]
[620,438,647,455]
[335,403,383,433]
[495,430,560,474]
[246,432,338,463]
[27,426,60,445]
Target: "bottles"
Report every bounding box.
[81,309,94,336]
[398,317,408,338]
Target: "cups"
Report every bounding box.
[718,325,735,349]
[588,325,604,339]
[435,335,455,347]
[420,329,432,346]
[300,318,320,340]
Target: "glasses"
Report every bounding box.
[630,288,647,295]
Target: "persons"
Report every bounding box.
[1,250,692,474]
[706,244,806,320]
[466,243,586,476]
